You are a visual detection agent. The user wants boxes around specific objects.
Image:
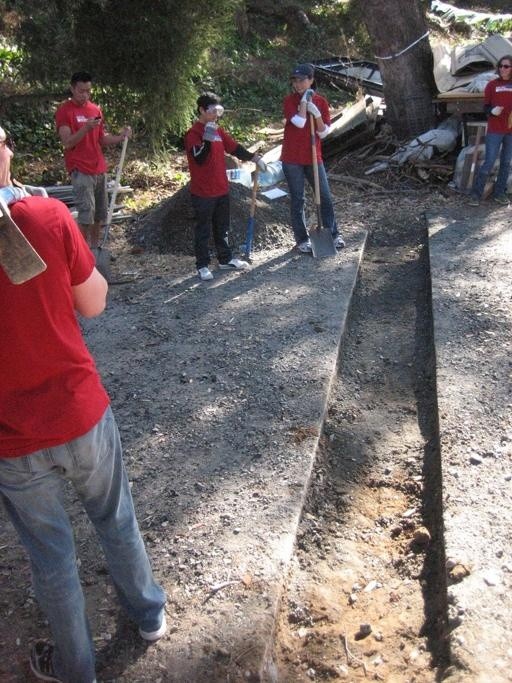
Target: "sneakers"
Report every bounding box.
[469,197,479,206]
[334,235,345,248]
[138,614,166,641]
[198,267,214,281]
[218,258,248,270]
[297,238,312,253]
[29,639,97,683]
[495,194,508,204]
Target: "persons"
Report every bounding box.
[52,71,133,256]
[468,54,511,209]
[179,91,267,281]
[0,125,170,680]
[279,62,346,254]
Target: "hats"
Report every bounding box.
[289,64,312,80]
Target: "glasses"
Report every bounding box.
[204,105,224,116]
[499,64,511,68]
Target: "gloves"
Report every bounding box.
[491,106,503,116]
[250,153,267,172]
[300,89,321,118]
[203,121,215,143]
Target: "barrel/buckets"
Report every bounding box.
[260,160,284,186]
[226,169,252,186]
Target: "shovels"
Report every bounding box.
[90,126,131,285]
[308,96,339,258]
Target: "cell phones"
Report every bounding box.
[95,117,101,120]
